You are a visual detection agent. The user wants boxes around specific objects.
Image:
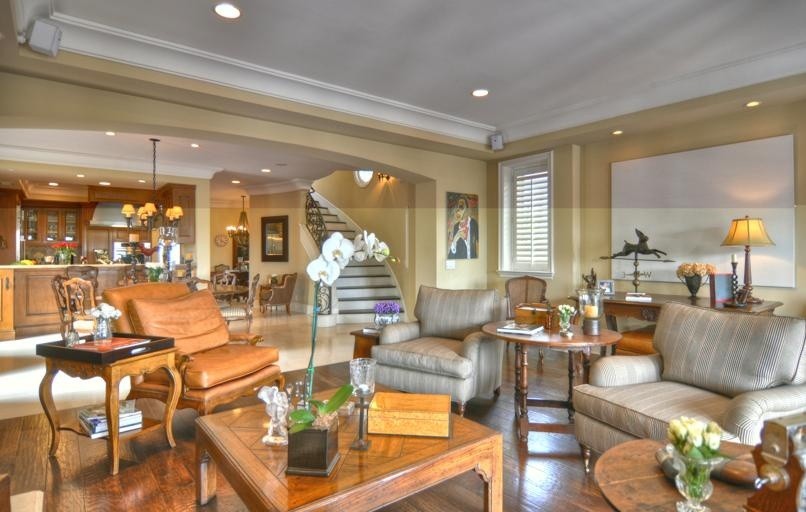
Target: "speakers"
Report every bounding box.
[27,17,62,57]
[490,135,503,150]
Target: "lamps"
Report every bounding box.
[720,215,776,304]
[376,172,392,184]
[225,194,251,250]
[118,138,184,240]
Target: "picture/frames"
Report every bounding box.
[596,280,616,296]
[259,214,290,263]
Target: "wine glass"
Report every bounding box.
[684,274,701,300]
[674,450,724,512]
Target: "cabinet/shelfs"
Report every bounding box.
[42,207,81,249]
[231,232,249,270]
[157,183,196,245]
[20,205,42,246]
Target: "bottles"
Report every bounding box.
[544,311,552,329]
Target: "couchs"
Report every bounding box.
[568,298,806,478]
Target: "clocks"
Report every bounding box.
[214,234,229,248]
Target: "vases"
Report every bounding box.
[57,252,72,265]
[284,410,342,479]
[374,310,401,330]
[670,448,729,512]
[683,274,704,300]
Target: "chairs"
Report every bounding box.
[100,279,285,422]
[48,257,300,342]
[0,472,49,512]
[364,284,511,418]
[505,274,549,356]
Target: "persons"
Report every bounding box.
[447,197,479,259]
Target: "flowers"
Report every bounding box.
[664,414,728,503]
[47,241,79,263]
[675,262,717,278]
[372,299,401,314]
[283,227,404,436]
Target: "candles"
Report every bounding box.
[128,233,139,244]
[732,253,738,263]
[584,303,599,320]
[184,254,192,260]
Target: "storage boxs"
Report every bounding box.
[511,301,563,330]
[364,389,456,442]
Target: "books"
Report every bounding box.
[72,337,151,354]
[79,405,142,439]
[497,321,544,335]
[625,292,651,302]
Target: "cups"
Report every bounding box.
[578,288,605,320]
[65,332,80,349]
[262,404,289,446]
[347,357,377,399]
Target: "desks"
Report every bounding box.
[566,290,786,359]
[35,330,184,476]
[349,326,381,360]
[480,317,622,443]
[591,432,799,512]
[0,261,152,340]
